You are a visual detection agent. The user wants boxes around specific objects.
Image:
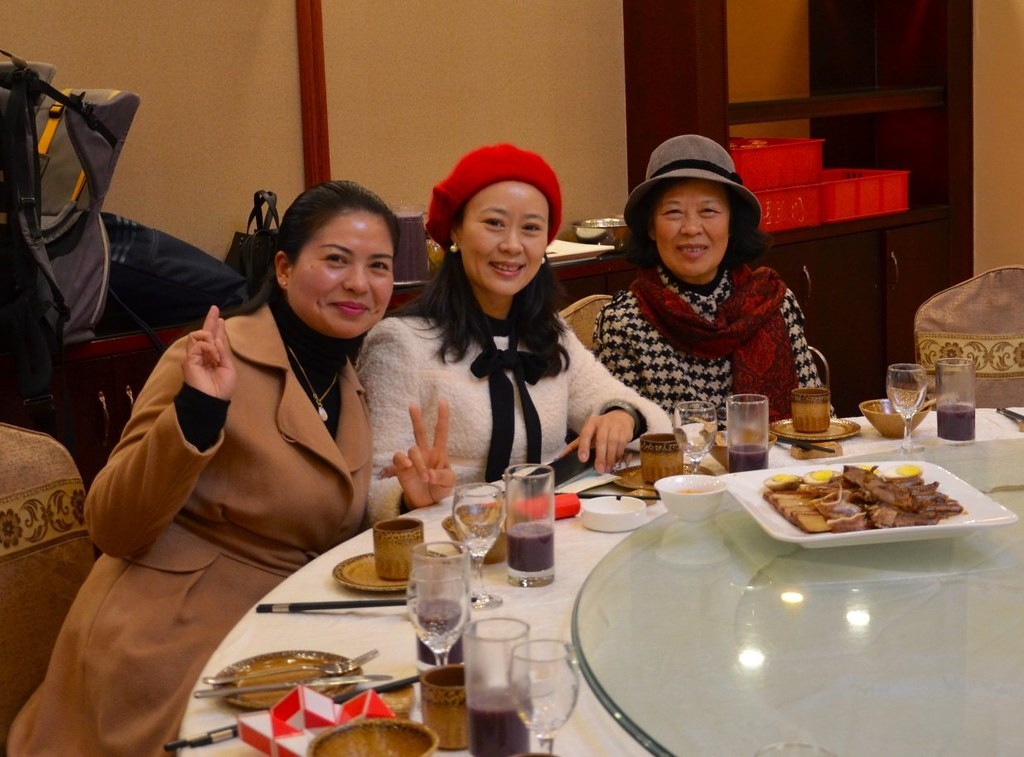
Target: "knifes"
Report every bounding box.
[192,674,394,700]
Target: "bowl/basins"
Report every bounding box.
[655,474,727,520]
[703,429,777,472]
[441,507,529,565]
[858,399,932,439]
[568,218,632,254]
[305,717,439,756]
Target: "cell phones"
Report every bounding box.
[527,447,597,486]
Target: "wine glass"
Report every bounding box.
[452,482,503,611]
[886,363,930,458]
[674,401,718,475]
[505,637,581,755]
[403,565,468,668]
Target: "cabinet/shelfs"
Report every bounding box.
[758,216,947,417]
[51,327,155,486]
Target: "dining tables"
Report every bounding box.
[174,406,1024,757]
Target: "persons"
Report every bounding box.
[0,179,402,757]
[354,140,671,527]
[585,134,839,428]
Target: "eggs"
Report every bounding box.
[765,474,801,489]
[801,470,842,485]
[853,464,882,478]
[882,465,924,479]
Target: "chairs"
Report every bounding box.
[0,425,94,757]
[915,264,1024,409]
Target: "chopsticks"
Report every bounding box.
[253,595,479,613]
[554,493,661,500]
[776,437,837,453]
[161,674,422,751]
[996,407,1024,422]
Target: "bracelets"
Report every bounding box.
[600,400,639,431]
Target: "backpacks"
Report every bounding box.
[0,50,141,372]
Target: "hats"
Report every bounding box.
[624,135,761,254]
[425,144,562,252]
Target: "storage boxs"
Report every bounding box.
[750,183,822,232]
[730,135,825,188]
[819,169,911,220]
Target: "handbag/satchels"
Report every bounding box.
[89,211,247,354]
[225,190,283,301]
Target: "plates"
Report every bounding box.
[768,417,862,440]
[715,460,1020,550]
[612,463,717,490]
[210,541,452,709]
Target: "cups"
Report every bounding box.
[372,512,580,757]
[505,462,555,588]
[424,231,446,279]
[639,356,976,484]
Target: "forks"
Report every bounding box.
[203,648,380,684]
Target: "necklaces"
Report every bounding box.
[286,345,341,423]
